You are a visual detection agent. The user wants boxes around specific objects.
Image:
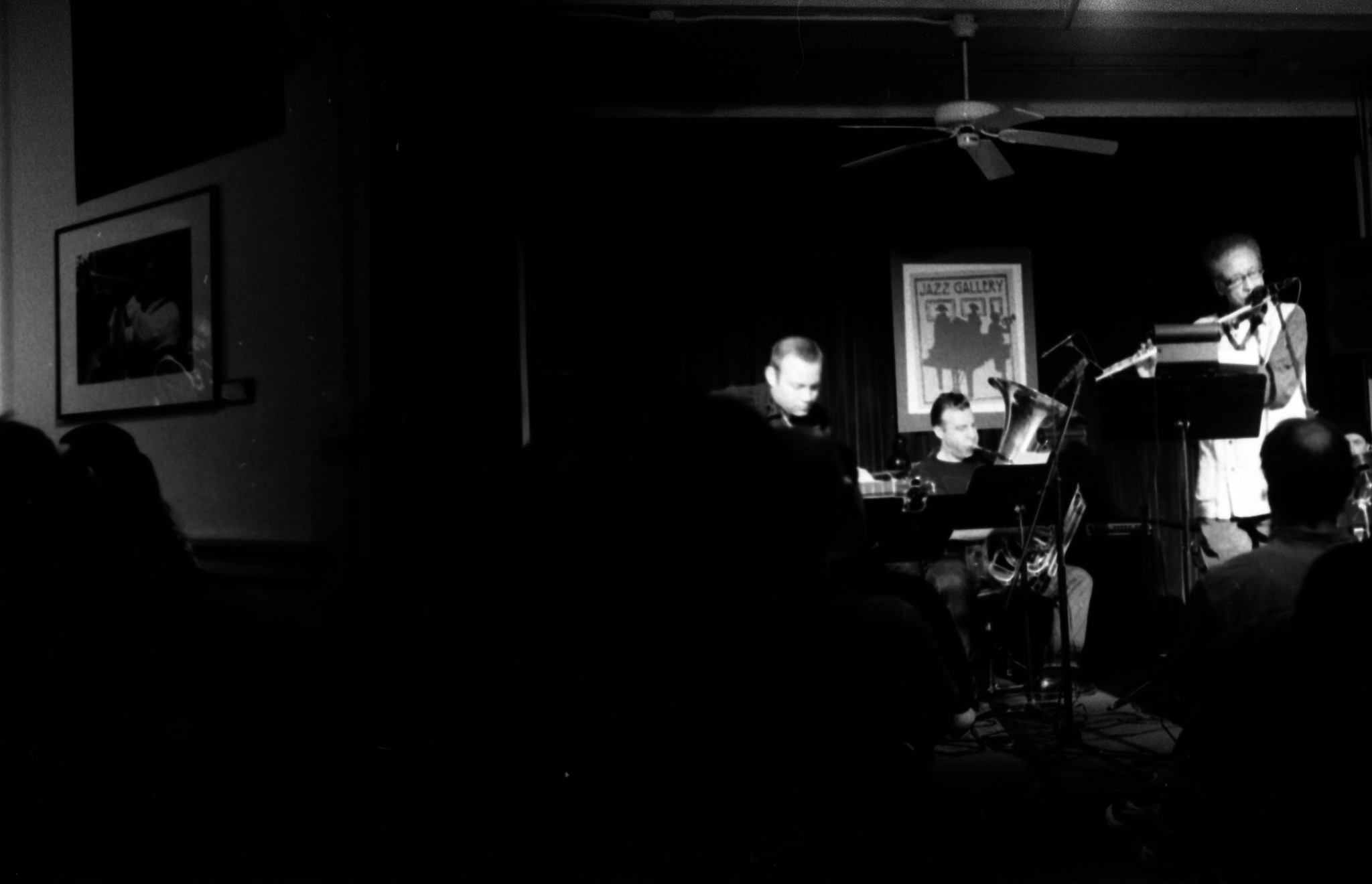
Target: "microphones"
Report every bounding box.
[970,443,1006,460]
[1251,278,1294,302]
[1059,357,1089,388]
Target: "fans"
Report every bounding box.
[841,13,1119,180]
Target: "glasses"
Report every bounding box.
[1220,268,1265,292]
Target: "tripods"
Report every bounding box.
[986,380,1159,753]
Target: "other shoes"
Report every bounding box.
[1072,674,1099,698]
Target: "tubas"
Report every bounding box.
[969,376,1088,589]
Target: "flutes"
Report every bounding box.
[1094,293,1274,383]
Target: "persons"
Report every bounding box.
[0,415,140,456]
[1183,420,1372,698]
[697,337,848,455]
[892,392,1099,727]
[1135,237,1309,570]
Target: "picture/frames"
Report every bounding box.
[54,185,225,422]
